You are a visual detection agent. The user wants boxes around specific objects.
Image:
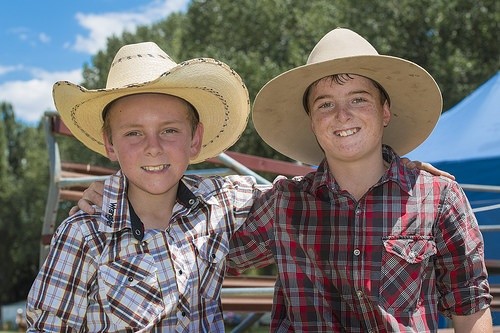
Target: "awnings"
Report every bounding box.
[397,69,500,328]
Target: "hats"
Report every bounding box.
[52,41,250,165]
[253,27,442,167]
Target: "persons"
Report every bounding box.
[25,42,456,333]
[67,27,496,333]
